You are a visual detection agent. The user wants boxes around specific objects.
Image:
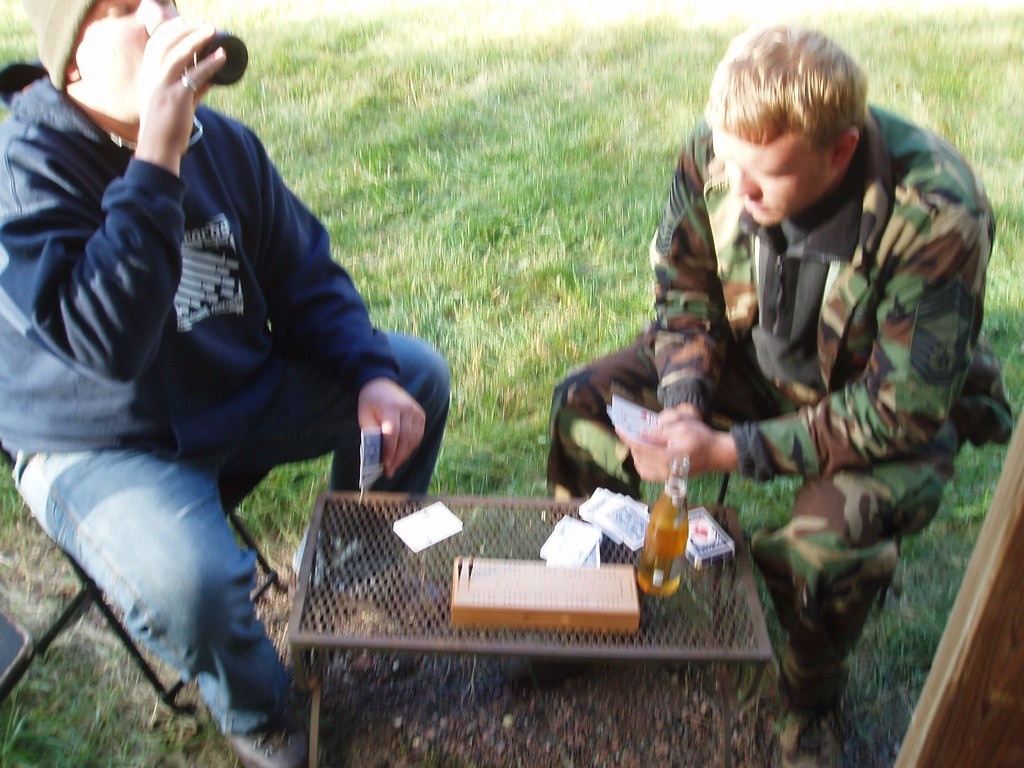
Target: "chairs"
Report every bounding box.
[0,444,285,727]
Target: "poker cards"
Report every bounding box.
[359,432,383,509]
[606,393,661,443]
[393,500,464,553]
[541,487,652,570]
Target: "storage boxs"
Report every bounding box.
[452,556,640,632]
[686,506,736,570]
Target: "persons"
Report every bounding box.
[0,0,451,768]
[500,23,1016,768]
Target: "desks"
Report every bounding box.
[284,490,772,768]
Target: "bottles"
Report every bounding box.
[637,453,692,598]
[192,30,250,88]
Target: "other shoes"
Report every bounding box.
[781,705,851,768]
[228,711,309,768]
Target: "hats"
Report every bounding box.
[27,0,177,92]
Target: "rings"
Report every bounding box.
[181,76,196,91]
